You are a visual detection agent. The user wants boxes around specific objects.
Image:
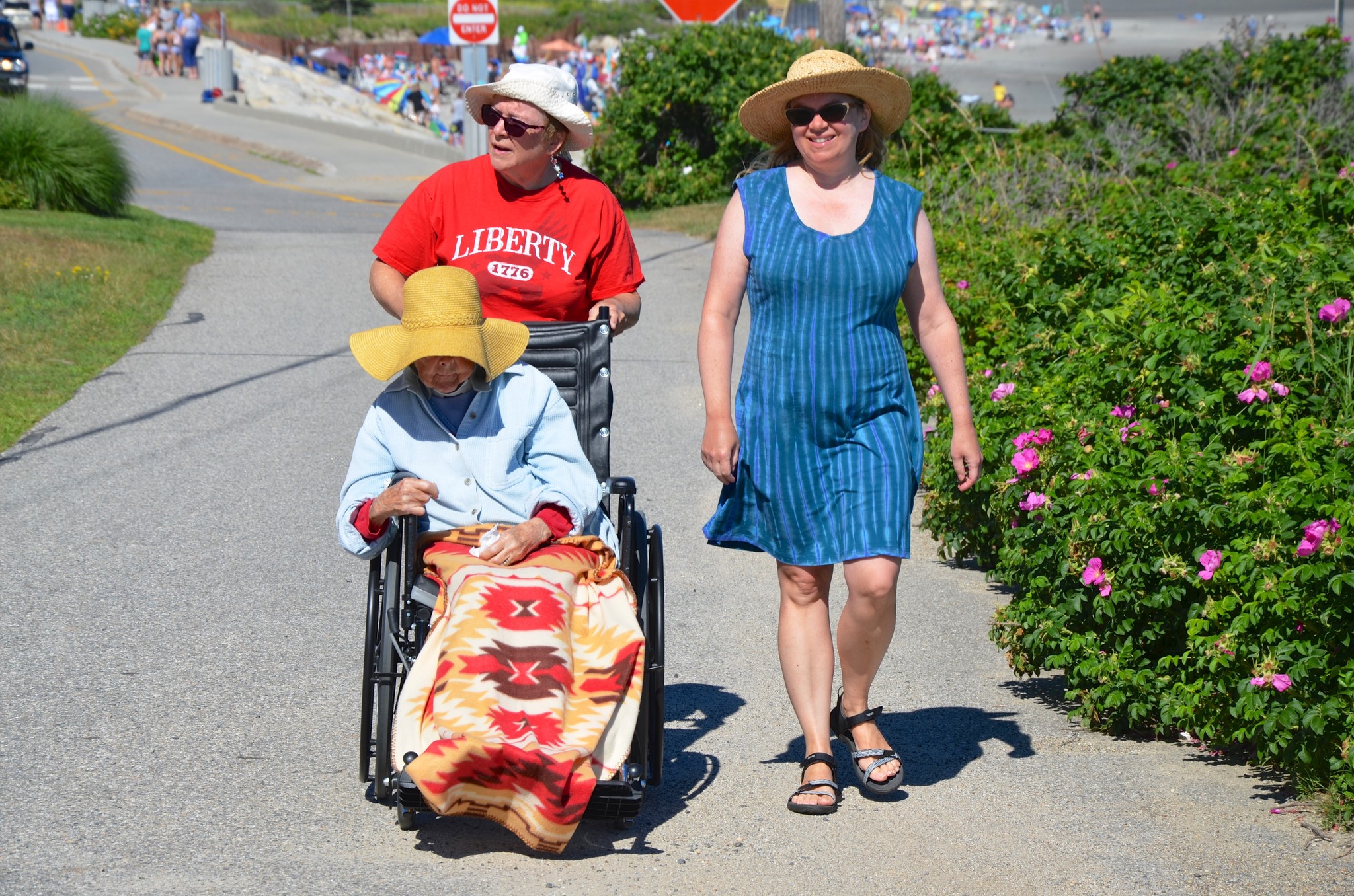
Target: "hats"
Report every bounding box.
[739,48,912,151]
[465,62,592,152]
[347,266,529,383]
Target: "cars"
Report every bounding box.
[0,14,34,97]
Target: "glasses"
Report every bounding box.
[481,104,547,137]
[785,101,861,127]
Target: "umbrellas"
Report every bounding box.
[308,45,351,67]
[537,38,579,52]
[419,28,450,45]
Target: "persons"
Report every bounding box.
[291,26,623,145]
[124,0,203,78]
[698,50,981,816]
[994,78,1014,111]
[336,266,645,854]
[28,0,78,35]
[369,63,644,336]
[762,0,1105,71]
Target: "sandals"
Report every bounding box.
[830,685,904,794]
[786,752,838,814]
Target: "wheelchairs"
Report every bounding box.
[359,305,664,832]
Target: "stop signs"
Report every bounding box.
[450,0,497,42]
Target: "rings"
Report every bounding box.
[502,559,511,567]
